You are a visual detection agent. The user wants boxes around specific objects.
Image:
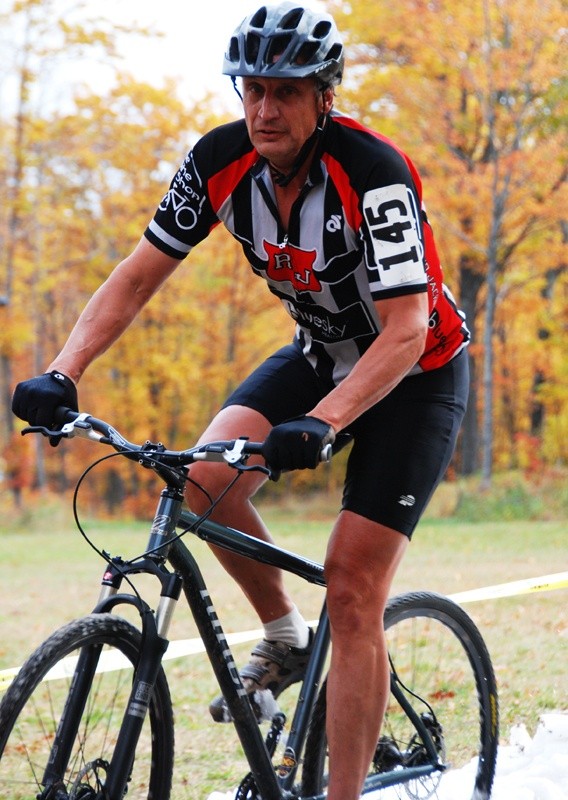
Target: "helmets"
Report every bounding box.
[221,1,345,82]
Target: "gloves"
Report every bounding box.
[262,413,336,482]
[12,371,80,447]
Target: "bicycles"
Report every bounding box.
[1,402,501,800]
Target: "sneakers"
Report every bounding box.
[208,626,319,722]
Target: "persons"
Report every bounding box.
[11,0,471,800]
